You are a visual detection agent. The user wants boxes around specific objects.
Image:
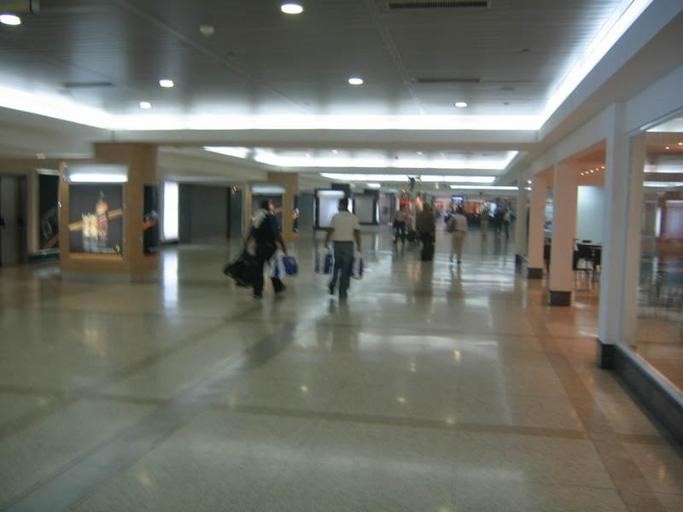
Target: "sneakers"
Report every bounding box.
[329,285,347,297]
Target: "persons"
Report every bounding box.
[241,200,288,300]
[415,202,435,260]
[445,206,468,264]
[391,205,409,244]
[441,200,514,240]
[323,197,363,299]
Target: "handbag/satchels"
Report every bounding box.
[314,247,332,274]
[225,253,261,287]
[268,255,298,277]
[447,216,455,232]
[352,249,363,279]
